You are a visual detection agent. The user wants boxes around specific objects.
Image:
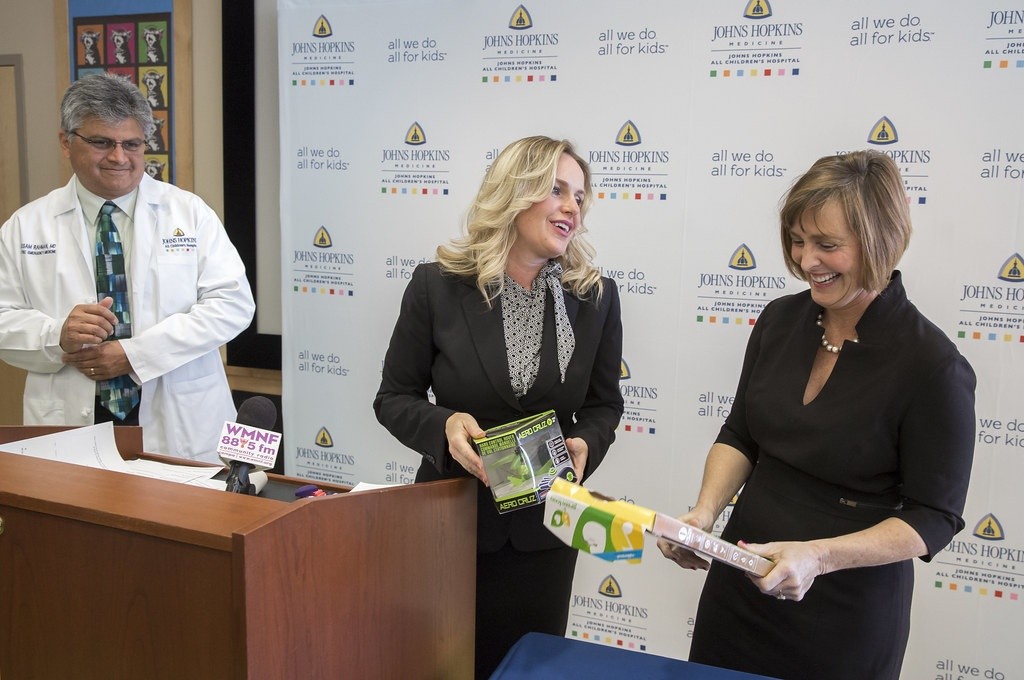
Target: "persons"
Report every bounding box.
[0,72,256,466]
[657,149,977,680]
[372,135,625,680]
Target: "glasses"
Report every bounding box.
[69,131,149,152]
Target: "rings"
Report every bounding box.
[91,368,95,374]
[777,590,785,600]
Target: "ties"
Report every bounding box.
[93,200,140,421]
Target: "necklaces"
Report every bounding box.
[816,314,859,354]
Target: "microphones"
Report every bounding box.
[216,396,281,493]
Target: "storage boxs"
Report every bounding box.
[542,477,775,580]
[472,410,578,515]
